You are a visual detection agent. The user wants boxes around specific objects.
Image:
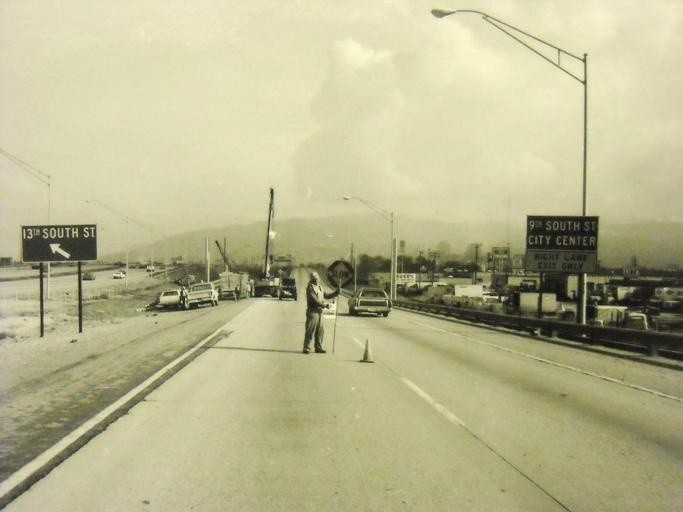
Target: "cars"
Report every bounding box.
[84,272,96,281]
[159,288,187,309]
[111,261,168,278]
[349,287,392,316]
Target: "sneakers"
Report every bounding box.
[303,346,326,355]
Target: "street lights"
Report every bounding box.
[472,241,483,285]
[343,195,397,304]
[433,7,589,331]
[430,251,440,274]
[419,251,424,288]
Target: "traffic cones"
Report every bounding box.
[358,337,376,364]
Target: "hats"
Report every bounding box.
[309,271,320,281]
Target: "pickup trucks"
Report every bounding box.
[187,281,220,307]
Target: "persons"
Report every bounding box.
[586,299,599,319]
[303,270,342,353]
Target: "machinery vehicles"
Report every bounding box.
[255,186,291,298]
[212,238,254,300]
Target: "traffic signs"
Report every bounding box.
[21,224,99,261]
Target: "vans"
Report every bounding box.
[275,278,297,301]
